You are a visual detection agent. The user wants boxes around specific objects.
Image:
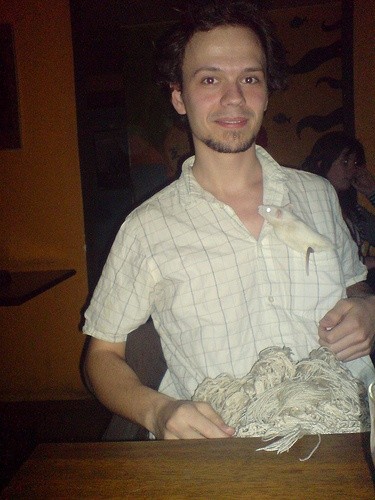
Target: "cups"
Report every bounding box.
[366,382,375,466]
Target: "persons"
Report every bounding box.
[80,7,375,440]
[299,133,375,284]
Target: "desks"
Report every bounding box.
[0,433,375,500]
[0,269,77,307]
